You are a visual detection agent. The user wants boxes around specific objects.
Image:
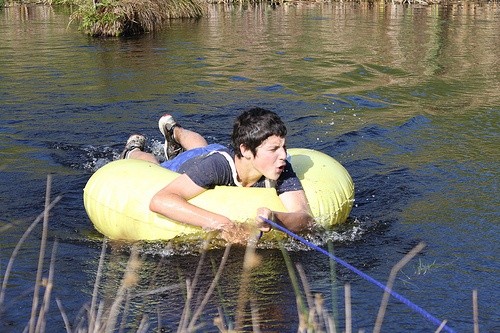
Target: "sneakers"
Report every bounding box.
[119,133,145,160]
[159,114,181,160]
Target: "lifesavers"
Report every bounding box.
[83,147,356,245]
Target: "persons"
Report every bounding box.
[119,106,314,243]
